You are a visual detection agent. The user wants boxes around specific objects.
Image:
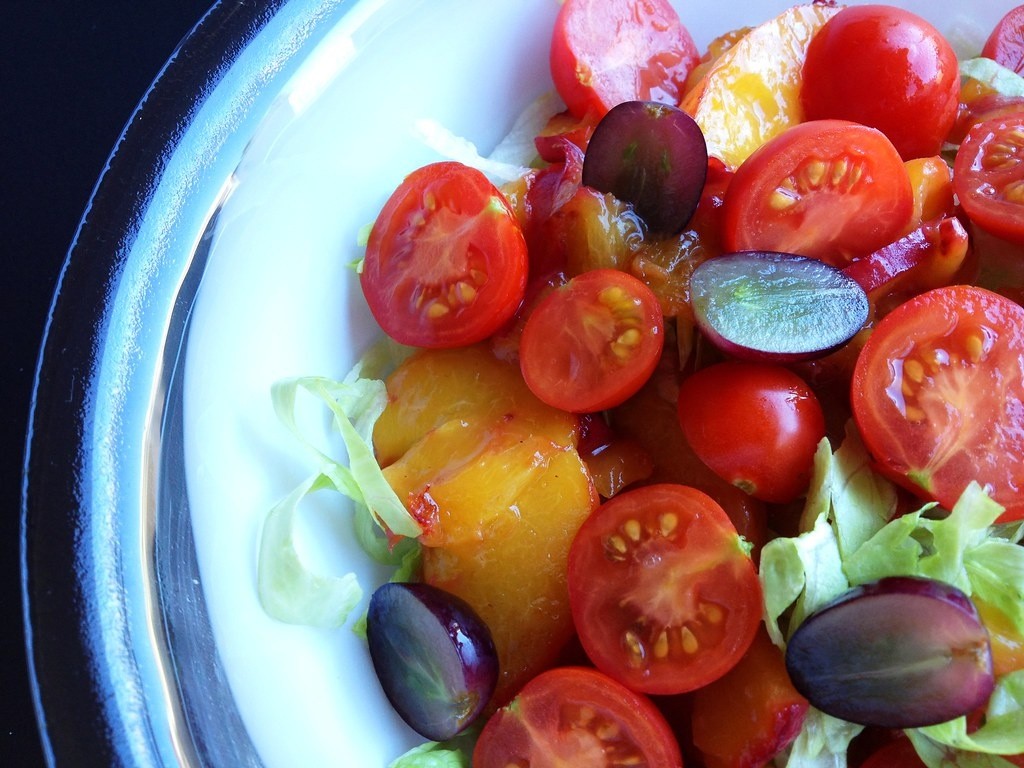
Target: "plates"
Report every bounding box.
[18,0,1024,768]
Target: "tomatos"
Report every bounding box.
[364,2,1022,768]
